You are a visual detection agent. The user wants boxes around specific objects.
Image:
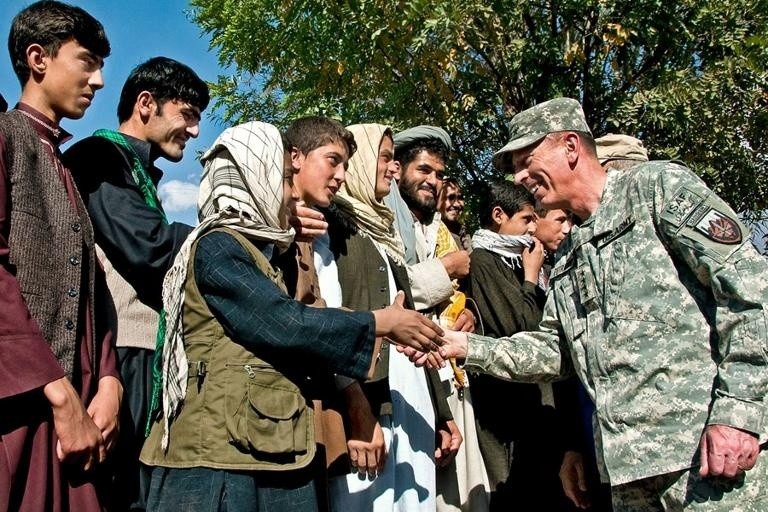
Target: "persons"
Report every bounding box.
[141,122,446,512]
[0,0,124,512]
[383,126,491,511]
[314,124,463,512]
[458,180,545,492]
[61,57,210,436]
[559,134,647,509]
[441,176,466,252]
[395,97,768,512]
[532,198,571,273]
[284,117,357,240]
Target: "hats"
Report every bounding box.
[492,97,593,173]
[594,134,648,165]
